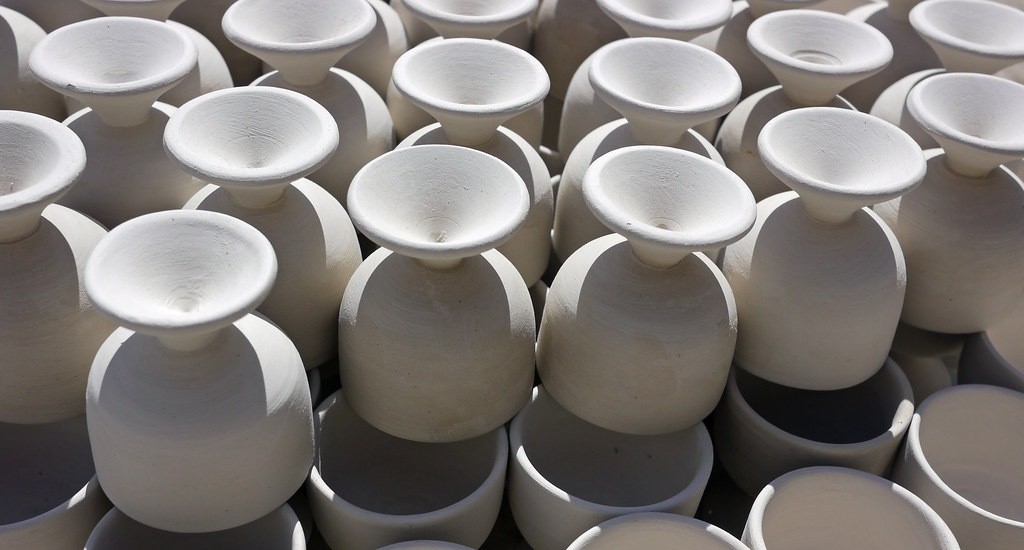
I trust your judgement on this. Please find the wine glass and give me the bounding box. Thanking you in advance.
[0,0,1024,550]
